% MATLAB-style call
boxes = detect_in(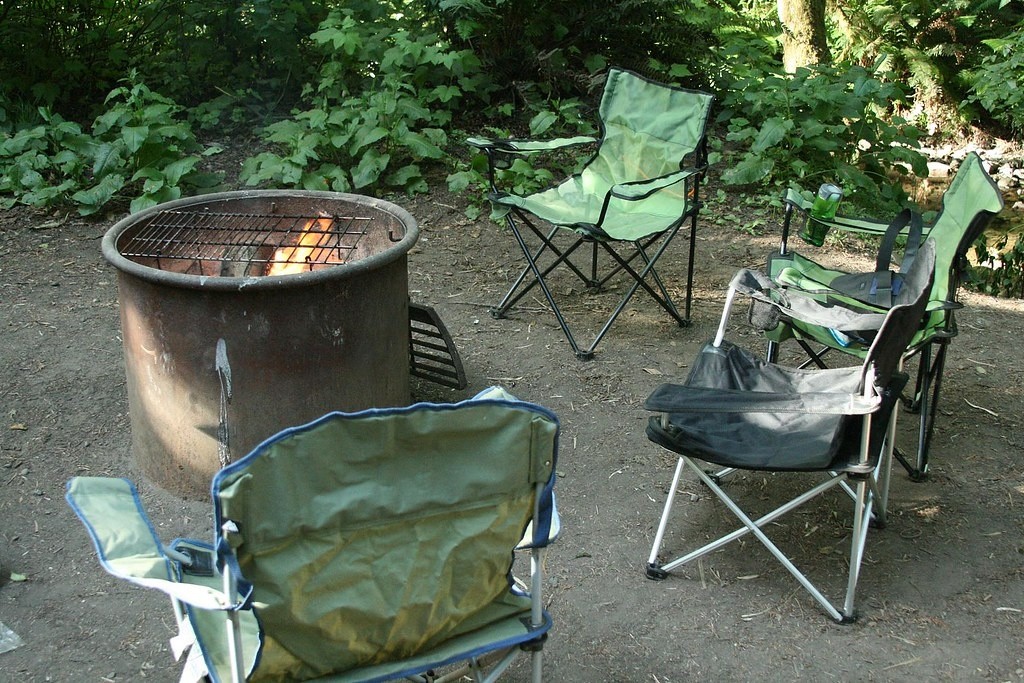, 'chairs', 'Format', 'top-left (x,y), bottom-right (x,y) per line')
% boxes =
(764, 153), (1003, 481)
(465, 65), (717, 360)
(66, 385), (563, 683)
(642, 236), (939, 624)
